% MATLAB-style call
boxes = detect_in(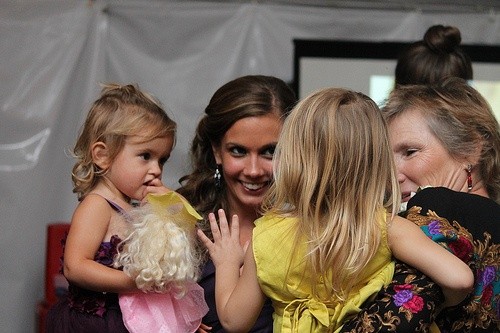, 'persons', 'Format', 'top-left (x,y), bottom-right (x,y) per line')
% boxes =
(395, 25), (473, 87)
(174, 75), (298, 333)
(197, 88), (473, 333)
(45, 82), (177, 333)
(337, 76), (500, 333)
